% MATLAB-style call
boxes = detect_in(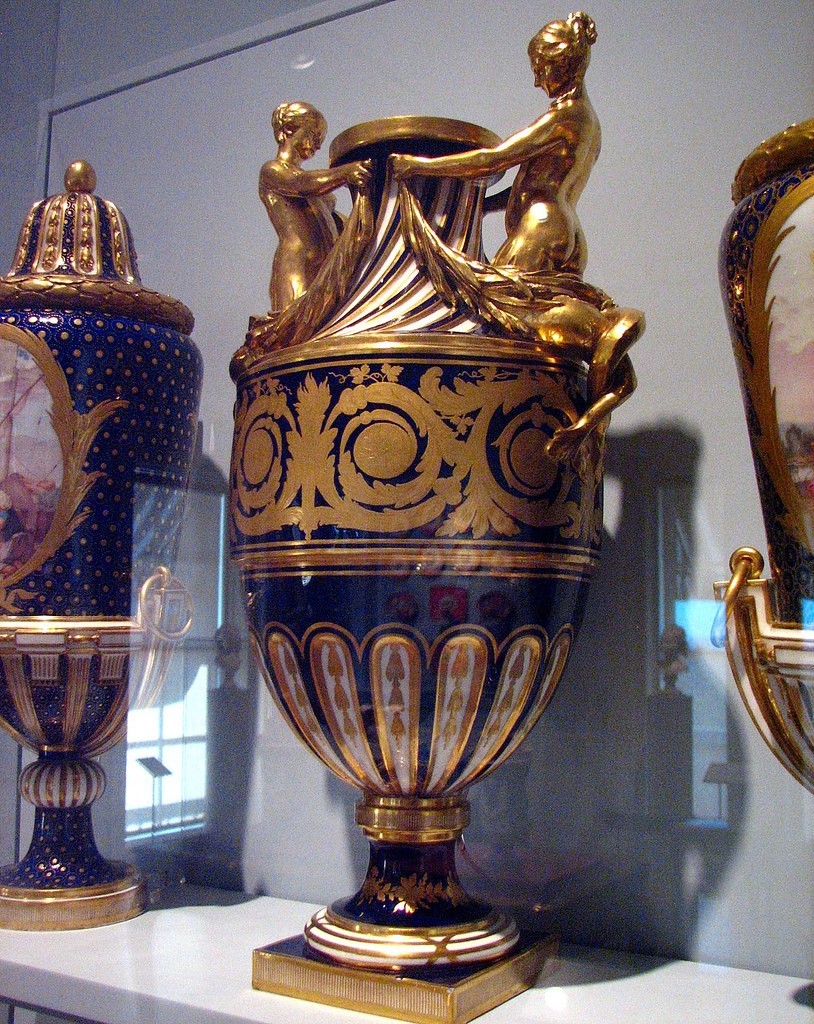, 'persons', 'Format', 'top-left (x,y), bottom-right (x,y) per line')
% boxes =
(388, 2), (646, 368)
(226, 100), (372, 384)
